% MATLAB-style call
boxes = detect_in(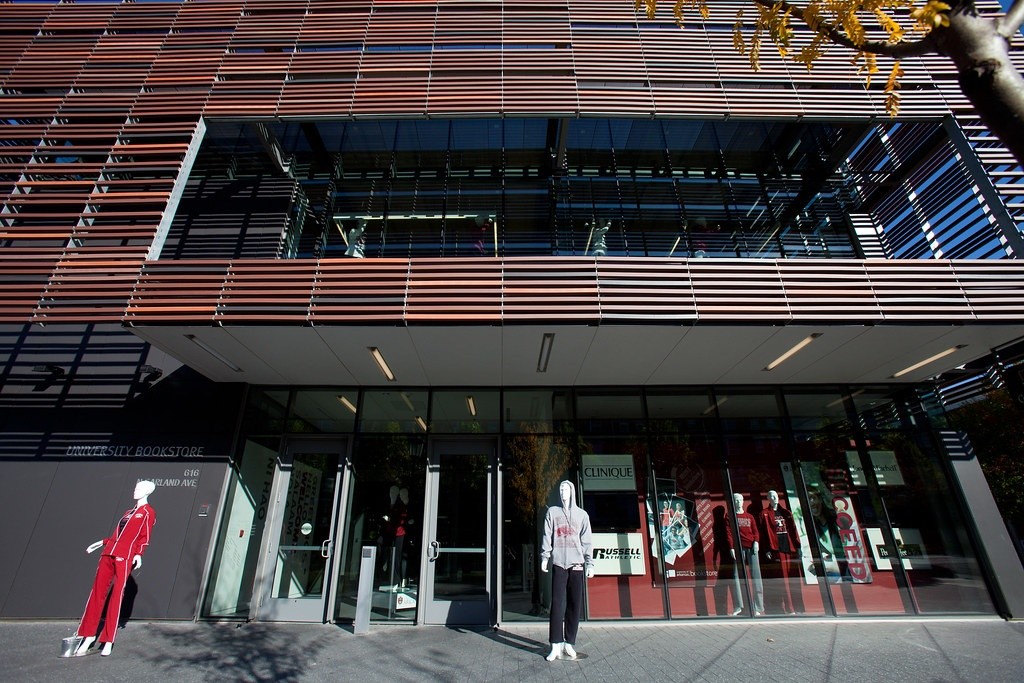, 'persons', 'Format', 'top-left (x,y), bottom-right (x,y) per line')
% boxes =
(724, 493), (764, 616)
(471, 217), (493, 255)
(759, 490), (801, 615)
(661, 500), (693, 555)
(344, 219), (368, 258)
(540, 479), (594, 661)
(591, 219), (611, 256)
(376, 486), (419, 588)
(803, 484), (849, 576)
(75, 480), (156, 656)
(692, 216), (720, 258)
